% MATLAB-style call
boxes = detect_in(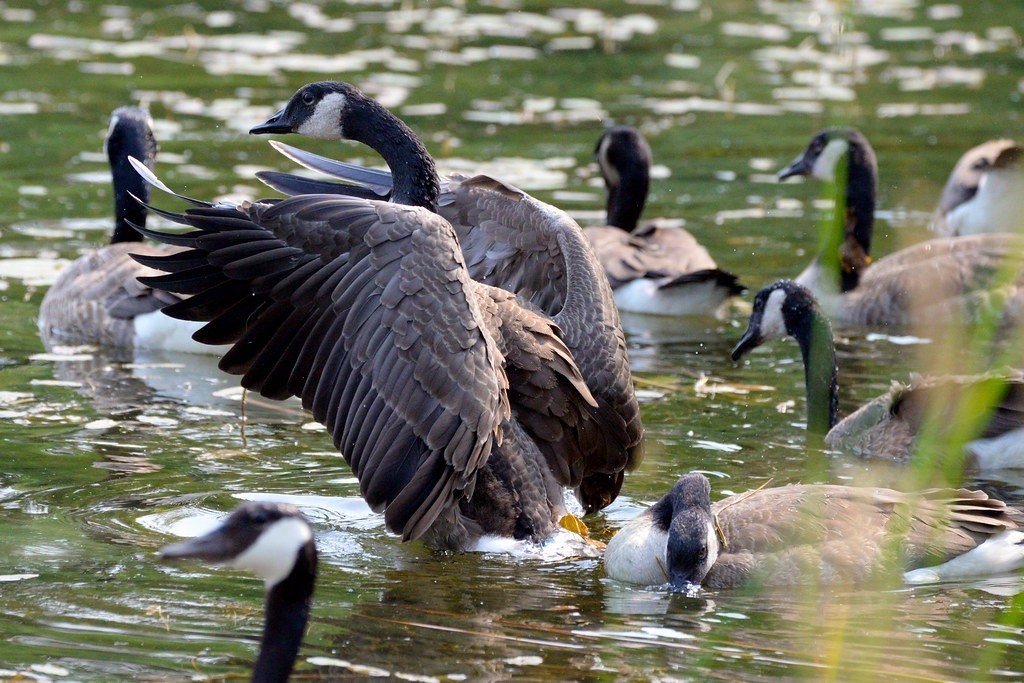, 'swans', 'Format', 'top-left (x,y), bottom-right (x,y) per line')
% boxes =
(159, 500), (320, 683)
(36, 79), (1024, 598)
